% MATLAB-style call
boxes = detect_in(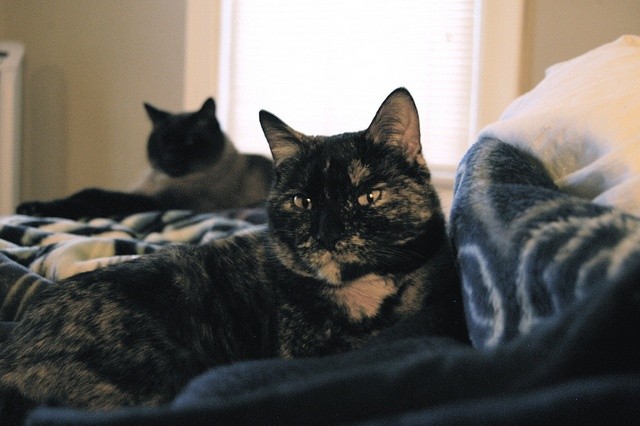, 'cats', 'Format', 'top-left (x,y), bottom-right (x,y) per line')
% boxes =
(134, 97), (273, 207)
(0, 87), (450, 423)
